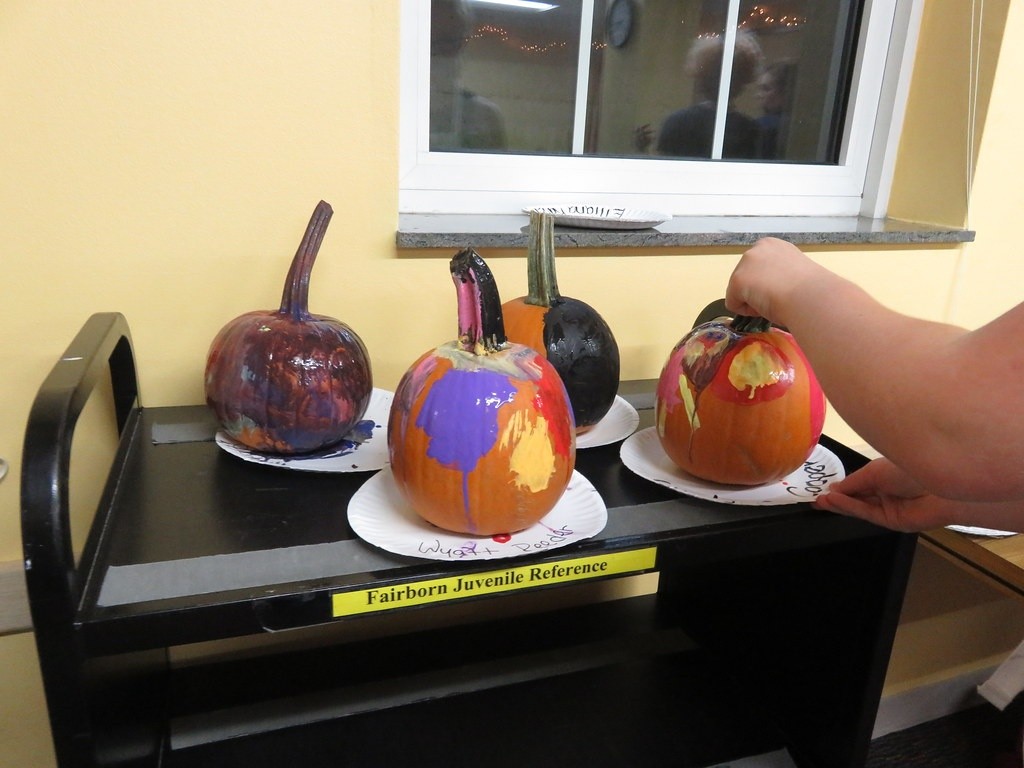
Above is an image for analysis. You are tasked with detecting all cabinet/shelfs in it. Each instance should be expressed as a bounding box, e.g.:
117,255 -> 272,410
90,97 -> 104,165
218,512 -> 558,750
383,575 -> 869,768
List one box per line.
14,310 -> 920,768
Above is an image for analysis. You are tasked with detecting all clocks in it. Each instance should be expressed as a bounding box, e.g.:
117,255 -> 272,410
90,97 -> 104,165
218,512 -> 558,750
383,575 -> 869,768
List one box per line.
606,0 -> 635,48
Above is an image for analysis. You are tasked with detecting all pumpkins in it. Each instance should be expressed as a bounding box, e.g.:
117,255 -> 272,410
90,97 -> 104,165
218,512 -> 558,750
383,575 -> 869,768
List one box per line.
654,313 -> 826,486
204,199 -> 372,454
386,244 -> 577,535
501,211 -> 620,437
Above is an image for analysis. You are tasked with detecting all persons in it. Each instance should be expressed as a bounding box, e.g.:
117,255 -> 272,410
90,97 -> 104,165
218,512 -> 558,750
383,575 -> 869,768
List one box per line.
726,235 -> 1024,534
460,87 -> 508,149
656,32 -> 788,159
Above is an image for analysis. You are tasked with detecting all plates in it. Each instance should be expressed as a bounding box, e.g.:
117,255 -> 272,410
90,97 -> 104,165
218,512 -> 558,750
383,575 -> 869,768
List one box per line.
620,425 -> 846,506
346,464 -> 609,562
523,201 -> 671,230
576,395 -> 640,448
215,387 -> 396,473
